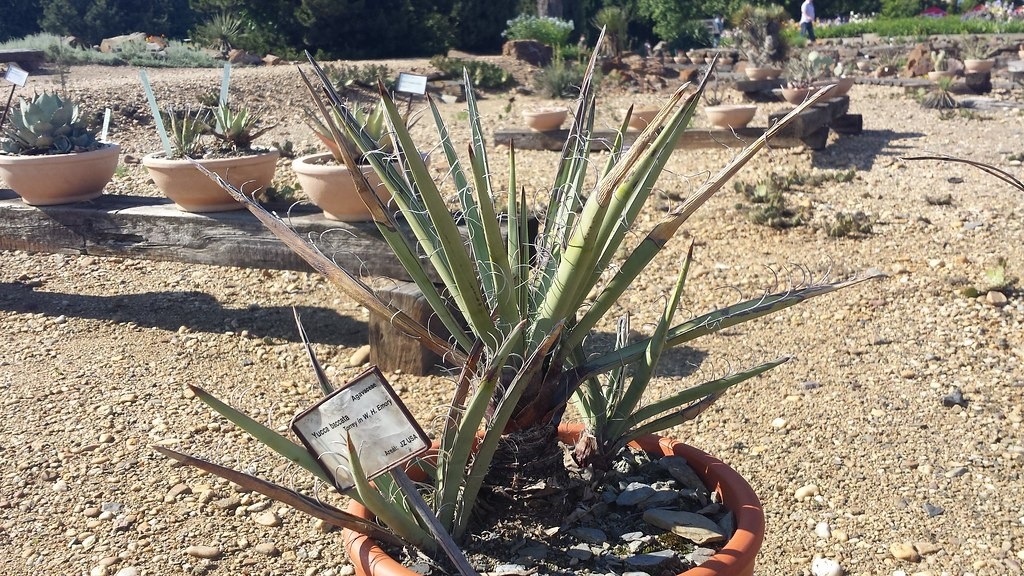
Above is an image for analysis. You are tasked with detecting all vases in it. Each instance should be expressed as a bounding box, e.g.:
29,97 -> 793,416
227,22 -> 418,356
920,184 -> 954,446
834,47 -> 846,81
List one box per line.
522,106 -> 568,131
705,105 -> 758,130
619,104 -> 661,131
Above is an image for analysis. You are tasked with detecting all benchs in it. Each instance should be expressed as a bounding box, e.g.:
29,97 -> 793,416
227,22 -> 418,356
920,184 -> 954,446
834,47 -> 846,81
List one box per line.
767,96 -> 863,150
0,188 -> 539,377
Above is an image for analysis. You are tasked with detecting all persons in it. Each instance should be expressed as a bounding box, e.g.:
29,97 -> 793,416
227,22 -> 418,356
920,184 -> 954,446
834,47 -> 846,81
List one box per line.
799,0 -> 816,44
712,14 -> 724,48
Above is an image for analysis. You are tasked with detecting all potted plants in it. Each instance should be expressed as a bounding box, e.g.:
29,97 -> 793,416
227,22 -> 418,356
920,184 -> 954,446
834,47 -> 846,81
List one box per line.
662,50 -> 732,65
745,47 -> 776,81
152,24 -> 890,576
808,51 -> 842,100
766,62 -> 783,80
962,36 -> 999,73
143,99 -> 279,212
832,66 -> 855,94
779,60 -> 823,108
0,89 -> 121,205
929,50 -> 955,83
291,92 -> 431,221
